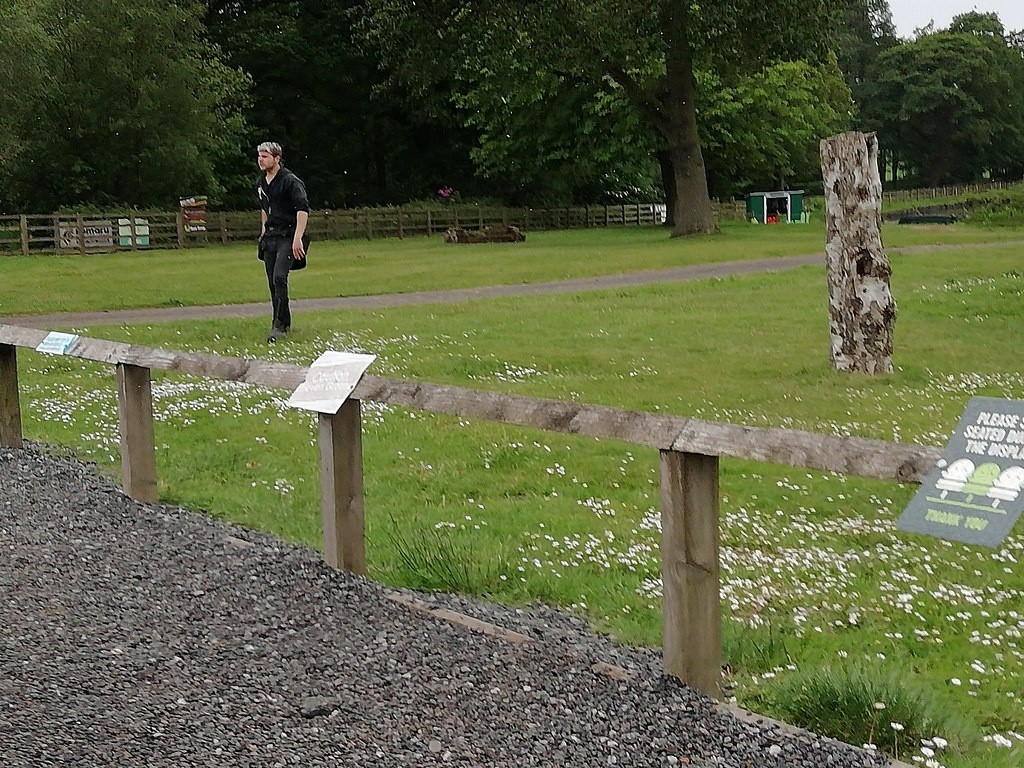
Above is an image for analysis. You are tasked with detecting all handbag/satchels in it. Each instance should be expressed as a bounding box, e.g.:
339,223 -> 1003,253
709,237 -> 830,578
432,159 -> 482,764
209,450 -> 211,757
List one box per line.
257,234 -> 311,270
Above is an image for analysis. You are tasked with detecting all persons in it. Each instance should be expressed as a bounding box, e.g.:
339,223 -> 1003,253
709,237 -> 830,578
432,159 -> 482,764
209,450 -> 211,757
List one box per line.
257,142 -> 308,343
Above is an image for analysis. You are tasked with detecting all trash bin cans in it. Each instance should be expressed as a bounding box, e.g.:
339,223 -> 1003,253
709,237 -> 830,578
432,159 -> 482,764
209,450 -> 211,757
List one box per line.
745,191 -> 804,224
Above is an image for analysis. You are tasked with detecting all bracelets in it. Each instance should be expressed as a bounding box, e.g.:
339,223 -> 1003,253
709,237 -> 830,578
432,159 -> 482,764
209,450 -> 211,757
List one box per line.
261,235 -> 263,238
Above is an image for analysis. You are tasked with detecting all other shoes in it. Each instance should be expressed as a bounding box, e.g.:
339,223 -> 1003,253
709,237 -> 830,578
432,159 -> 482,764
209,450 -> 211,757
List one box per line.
266,329 -> 287,338
